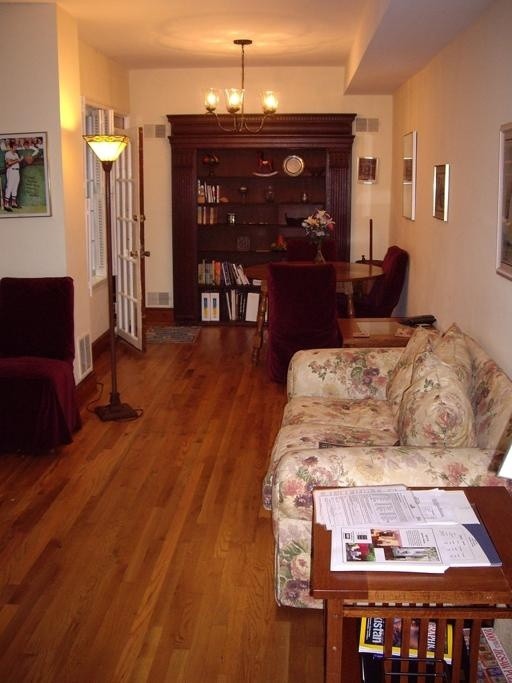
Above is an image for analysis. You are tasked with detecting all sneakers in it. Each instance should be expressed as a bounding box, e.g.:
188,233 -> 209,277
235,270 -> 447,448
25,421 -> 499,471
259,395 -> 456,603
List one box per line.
4,207 -> 13,212
12,204 -> 22,209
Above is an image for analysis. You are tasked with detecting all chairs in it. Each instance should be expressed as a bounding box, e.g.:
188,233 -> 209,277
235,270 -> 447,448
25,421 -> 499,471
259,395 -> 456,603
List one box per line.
264,263 -> 338,379
0,276 -> 83,457
359,245 -> 408,319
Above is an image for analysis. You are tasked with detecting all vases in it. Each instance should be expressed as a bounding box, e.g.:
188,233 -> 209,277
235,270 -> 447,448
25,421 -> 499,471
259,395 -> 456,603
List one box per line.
313,241 -> 325,262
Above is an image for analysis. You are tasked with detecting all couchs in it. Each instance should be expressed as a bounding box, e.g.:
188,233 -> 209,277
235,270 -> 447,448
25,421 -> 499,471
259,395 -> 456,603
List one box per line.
260,323 -> 511,609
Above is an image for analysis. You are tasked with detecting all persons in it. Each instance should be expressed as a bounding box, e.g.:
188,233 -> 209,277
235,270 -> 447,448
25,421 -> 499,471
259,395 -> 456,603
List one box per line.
0,137 -> 43,212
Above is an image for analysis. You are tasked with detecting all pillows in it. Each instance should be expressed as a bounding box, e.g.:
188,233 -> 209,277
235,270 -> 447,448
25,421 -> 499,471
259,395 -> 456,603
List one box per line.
431,322 -> 472,388
398,351 -> 478,449
385,326 -> 441,415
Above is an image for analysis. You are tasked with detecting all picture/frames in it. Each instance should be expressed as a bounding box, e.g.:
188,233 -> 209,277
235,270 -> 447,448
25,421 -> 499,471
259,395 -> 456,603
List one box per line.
494,122 -> 512,280
401,131 -> 416,222
0,131 -> 52,219
433,164 -> 449,223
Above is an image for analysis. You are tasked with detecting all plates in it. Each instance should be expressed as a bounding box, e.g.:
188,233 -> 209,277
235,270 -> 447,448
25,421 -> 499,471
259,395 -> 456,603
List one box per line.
283,156 -> 304,177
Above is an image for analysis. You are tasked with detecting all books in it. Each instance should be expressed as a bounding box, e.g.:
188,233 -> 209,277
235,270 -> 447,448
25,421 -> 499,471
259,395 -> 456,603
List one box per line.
358,614 -> 512,682
394,327 -> 441,338
329,523 -> 502,575
197,178 -> 226,203
197,205 -> 218,224
225,288 -> 247,321
197,258 -> 250,286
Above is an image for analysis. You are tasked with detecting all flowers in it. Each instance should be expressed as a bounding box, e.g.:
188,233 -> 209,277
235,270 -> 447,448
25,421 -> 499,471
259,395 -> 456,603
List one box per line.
301,208 -> 336,241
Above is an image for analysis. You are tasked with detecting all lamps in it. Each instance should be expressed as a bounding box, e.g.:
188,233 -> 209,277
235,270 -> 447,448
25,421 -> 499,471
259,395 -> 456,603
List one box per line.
85,133 -> 139,424
203,39 -> 279,136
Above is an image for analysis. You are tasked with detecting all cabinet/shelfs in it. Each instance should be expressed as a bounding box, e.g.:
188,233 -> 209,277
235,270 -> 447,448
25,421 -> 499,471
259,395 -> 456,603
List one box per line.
164,110 -> 356,325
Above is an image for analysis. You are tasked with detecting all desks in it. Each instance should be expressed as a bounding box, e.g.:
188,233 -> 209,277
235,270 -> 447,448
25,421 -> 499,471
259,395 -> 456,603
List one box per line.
242,261 -> 383,367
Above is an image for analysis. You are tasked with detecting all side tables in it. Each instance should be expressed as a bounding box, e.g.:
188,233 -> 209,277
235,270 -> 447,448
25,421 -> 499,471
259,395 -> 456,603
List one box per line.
310,484 -> 512,683
337,317 -> 442,348
